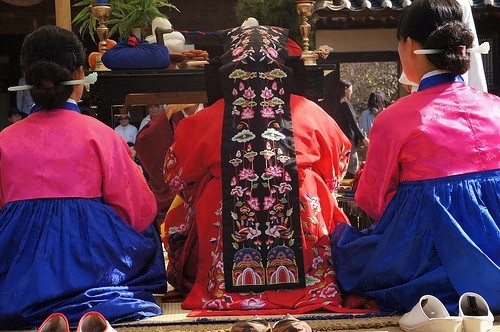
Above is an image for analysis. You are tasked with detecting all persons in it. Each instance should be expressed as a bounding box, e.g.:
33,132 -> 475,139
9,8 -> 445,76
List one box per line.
0,25 -> 164,324
115,103 -> 160,182
157,24 -> 376,317
340,80 -> 391,177
7,73 -> 33,125
328,0 -> 500,313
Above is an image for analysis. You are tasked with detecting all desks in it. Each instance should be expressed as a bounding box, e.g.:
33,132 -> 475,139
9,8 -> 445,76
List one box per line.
87,64 -> 337,129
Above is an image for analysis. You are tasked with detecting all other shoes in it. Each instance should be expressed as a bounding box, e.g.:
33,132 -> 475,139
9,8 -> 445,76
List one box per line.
37,313 -> 70,332
459,292 -> 494,332
271,313 -> 311,332
399,294 -> 464,332
231,315 -> 270,332
76,311 -> 117,332
